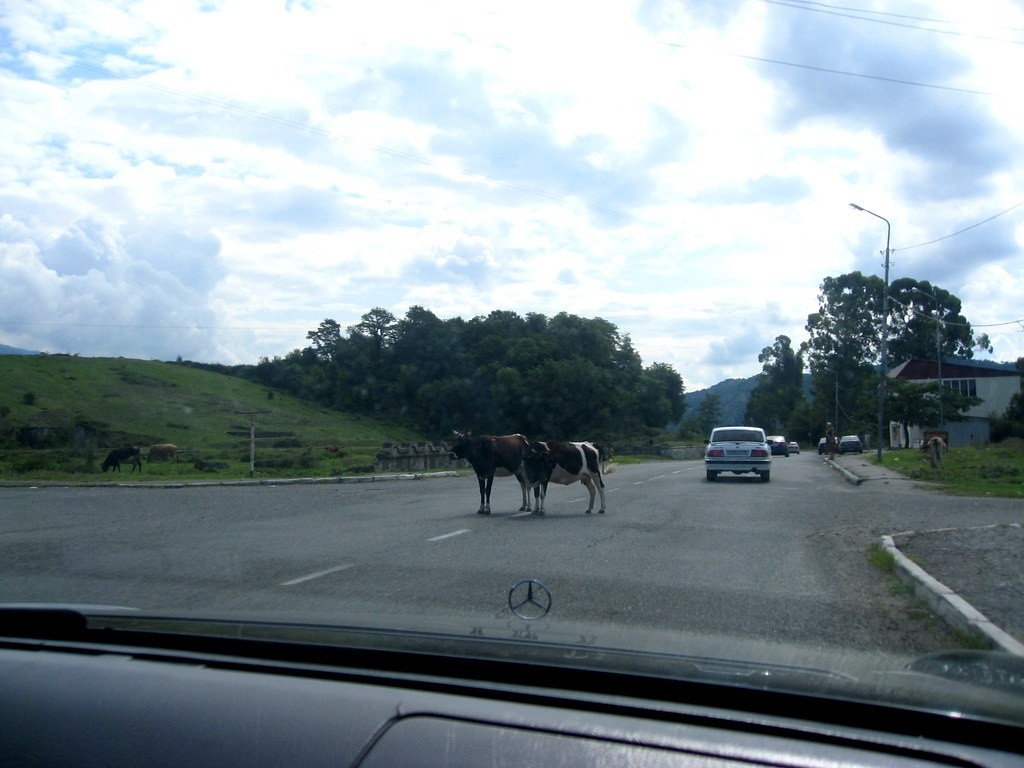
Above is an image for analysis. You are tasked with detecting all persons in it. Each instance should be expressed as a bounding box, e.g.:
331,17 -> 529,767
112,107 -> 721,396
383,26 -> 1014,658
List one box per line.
825,421 -> 837,460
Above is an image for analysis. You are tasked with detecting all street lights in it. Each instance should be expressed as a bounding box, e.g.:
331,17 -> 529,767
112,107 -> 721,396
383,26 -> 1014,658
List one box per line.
849,202 -> 890,465
802,395 -> 812,449
912,287 -> 944,429
234,409 -> 270,478
824,367 -> 839,446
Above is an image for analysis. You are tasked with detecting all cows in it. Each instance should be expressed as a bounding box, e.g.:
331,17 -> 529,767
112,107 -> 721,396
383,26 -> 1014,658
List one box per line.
193,461 -> 231,473
926,436 -> 946,469
100,447 -> 143,475
450,427 -> 615,516
146,444 -> 178,464
325,446 -> 338,454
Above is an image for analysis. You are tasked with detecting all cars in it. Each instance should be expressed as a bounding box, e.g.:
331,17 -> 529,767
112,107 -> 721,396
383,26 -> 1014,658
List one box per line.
703,426 -> 774,481
766,435 -> 790,457
818,437 -> 840,455
838,435 -> 863,454
788,442 -> 800,454
